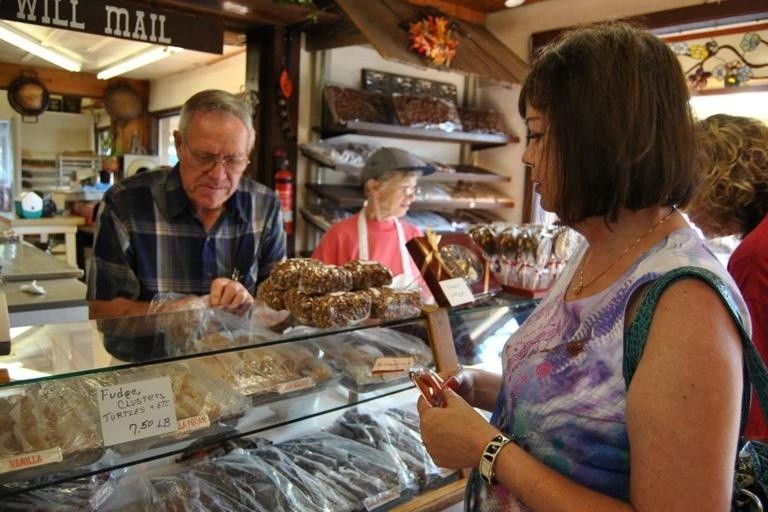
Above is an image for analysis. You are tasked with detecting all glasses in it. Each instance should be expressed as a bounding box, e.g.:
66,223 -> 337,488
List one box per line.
190,155 -> 251,172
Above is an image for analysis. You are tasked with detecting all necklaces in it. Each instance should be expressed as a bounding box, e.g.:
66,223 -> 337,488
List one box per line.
572,209 -> 675,294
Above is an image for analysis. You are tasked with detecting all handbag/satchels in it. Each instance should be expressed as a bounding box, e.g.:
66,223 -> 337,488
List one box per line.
738,434 -> 760,447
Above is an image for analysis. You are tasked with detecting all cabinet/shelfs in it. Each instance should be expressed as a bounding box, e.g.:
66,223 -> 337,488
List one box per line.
300,122 -> 520,257
1,289 -> 541,512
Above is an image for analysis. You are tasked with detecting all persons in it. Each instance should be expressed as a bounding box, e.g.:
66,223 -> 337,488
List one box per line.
410,25 -> 751,511
678,115 -> 768,443
85,89 -> 287,320
310,147 -> 436,291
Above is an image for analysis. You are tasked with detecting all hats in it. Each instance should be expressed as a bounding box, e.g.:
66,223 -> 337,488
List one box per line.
362,148 -> 436,183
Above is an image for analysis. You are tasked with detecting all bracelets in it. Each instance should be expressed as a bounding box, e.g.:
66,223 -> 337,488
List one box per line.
479,430 -> 522,485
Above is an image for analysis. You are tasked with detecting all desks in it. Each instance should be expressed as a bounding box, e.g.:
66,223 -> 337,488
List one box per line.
0,211 -> 86,265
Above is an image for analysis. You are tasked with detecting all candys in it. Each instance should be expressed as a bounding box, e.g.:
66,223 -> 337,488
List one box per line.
440,221 -> 576,289
